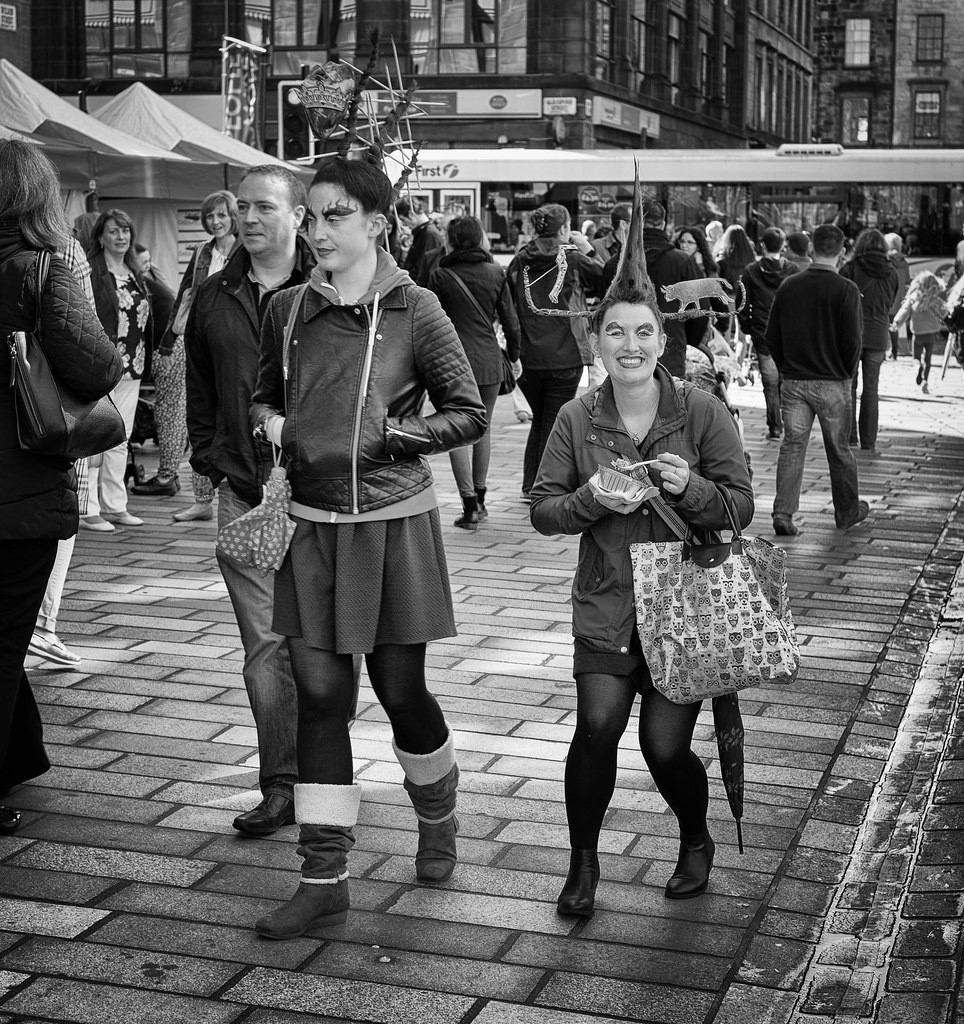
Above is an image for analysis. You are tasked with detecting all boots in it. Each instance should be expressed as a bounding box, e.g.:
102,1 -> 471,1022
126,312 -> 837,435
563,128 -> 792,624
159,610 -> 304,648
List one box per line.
254,783 -> 363,939
392,720 -> 460,881
475,486 -> 487,517
454,496 -> 479,530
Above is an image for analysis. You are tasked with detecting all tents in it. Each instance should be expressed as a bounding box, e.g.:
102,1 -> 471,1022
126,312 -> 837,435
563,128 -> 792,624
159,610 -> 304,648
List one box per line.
0,43 -> 319,201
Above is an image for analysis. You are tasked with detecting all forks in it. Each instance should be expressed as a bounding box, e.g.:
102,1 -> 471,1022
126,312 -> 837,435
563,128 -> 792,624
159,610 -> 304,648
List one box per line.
611,453 -> 680,470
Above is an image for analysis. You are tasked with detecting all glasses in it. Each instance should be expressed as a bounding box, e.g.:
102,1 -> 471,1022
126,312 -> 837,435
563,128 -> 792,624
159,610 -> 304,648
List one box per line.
678,239 -> 697,244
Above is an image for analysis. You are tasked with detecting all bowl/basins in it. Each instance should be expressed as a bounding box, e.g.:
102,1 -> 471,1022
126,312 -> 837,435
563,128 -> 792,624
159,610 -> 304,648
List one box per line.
595,463 -> 649,499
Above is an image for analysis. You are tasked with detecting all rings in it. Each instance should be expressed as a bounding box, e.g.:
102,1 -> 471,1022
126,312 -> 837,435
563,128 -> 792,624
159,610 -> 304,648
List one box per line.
674,467 -> 677,475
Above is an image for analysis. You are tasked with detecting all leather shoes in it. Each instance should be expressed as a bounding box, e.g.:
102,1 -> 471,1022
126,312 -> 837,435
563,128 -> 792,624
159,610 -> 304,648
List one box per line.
557,848 -> 600,916
232,794 -> 296,833
665,829 -> 715,900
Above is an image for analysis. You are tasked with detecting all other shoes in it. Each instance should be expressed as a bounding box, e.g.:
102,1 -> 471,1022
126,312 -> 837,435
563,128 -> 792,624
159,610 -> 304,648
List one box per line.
520,493 -> 532,502
773,519 -> 797,536
100,513 -> 144,525
27,630 -> 82,665
766,431 -> 780,441
79,518 -> 115,531
836,501 -> 869,528
173,506 -> 213,521
0,808 -> 21,829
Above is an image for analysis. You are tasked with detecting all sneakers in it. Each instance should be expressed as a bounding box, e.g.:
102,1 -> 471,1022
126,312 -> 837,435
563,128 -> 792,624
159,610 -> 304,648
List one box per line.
174,476 -> 181,490
132,478 -> 177,495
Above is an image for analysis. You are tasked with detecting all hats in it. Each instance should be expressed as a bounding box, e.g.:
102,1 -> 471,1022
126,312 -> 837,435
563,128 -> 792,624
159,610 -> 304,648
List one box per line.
884,232 -> 901,248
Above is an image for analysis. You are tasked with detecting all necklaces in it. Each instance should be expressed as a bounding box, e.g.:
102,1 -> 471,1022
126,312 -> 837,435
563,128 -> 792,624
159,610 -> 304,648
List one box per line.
617,397 -> 659,446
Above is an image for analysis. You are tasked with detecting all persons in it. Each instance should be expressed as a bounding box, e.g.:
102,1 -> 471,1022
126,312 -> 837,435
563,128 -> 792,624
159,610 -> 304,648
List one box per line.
56,188 -> 964,530
183,30 -> 489,940
531,156 -> 803,922
0,133 -> 124,840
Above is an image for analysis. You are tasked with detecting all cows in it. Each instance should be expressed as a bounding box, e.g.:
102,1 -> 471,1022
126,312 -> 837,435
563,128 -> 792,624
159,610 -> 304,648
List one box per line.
661,278 -> 736,313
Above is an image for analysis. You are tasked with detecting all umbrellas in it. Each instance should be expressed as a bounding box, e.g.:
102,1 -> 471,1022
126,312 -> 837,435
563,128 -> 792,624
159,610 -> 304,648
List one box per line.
215,467 -> 297,578
712,483 -> 746,855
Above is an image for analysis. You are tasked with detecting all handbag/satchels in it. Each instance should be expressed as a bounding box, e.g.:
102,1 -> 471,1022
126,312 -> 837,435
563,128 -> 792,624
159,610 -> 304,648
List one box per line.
629,487 -> 800,705
171,241 -> 211,335
497,348 -> 515,395
7,247 -> 127,458
